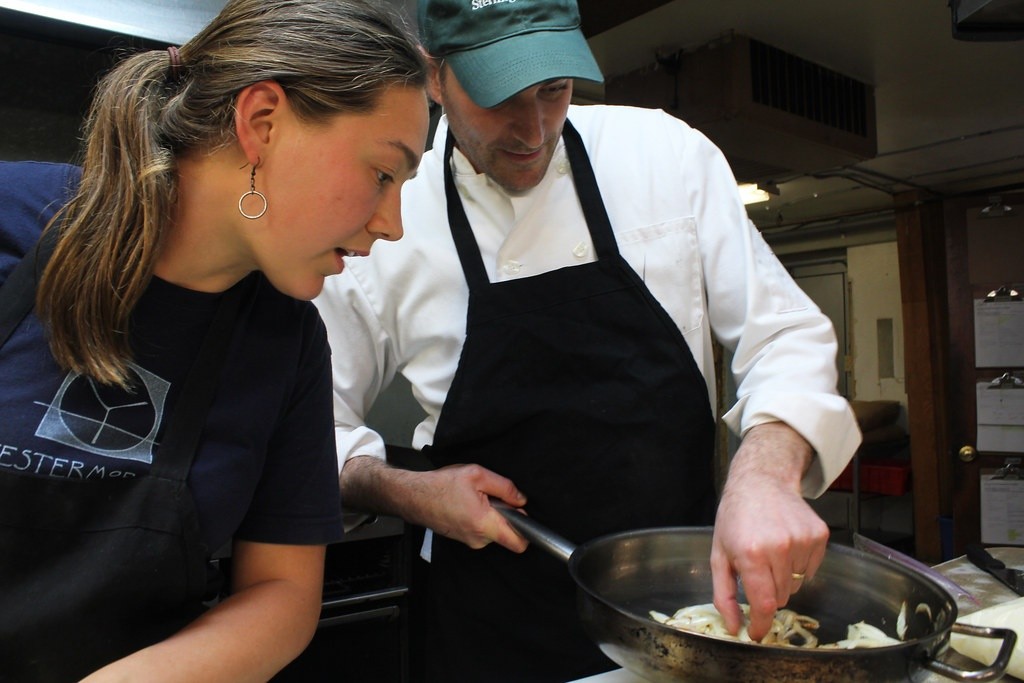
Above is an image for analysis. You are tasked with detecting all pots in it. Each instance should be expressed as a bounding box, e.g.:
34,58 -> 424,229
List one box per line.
488,495 -> 1018,683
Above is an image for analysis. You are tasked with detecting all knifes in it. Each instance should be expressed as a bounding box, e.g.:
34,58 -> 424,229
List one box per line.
966,546 -> 1024,597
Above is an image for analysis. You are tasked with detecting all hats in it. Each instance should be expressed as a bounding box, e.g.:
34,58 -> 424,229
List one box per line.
416,1 -> 605,103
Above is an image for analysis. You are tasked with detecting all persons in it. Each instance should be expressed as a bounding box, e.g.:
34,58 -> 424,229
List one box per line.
1,0 -> 431,683
311,1 -> 863,683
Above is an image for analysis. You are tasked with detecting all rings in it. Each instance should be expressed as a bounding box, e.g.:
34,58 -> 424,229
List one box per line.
791,572 -> 806,579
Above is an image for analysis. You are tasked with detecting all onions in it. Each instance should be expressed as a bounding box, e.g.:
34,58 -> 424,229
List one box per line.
650,600 -> 909,648
